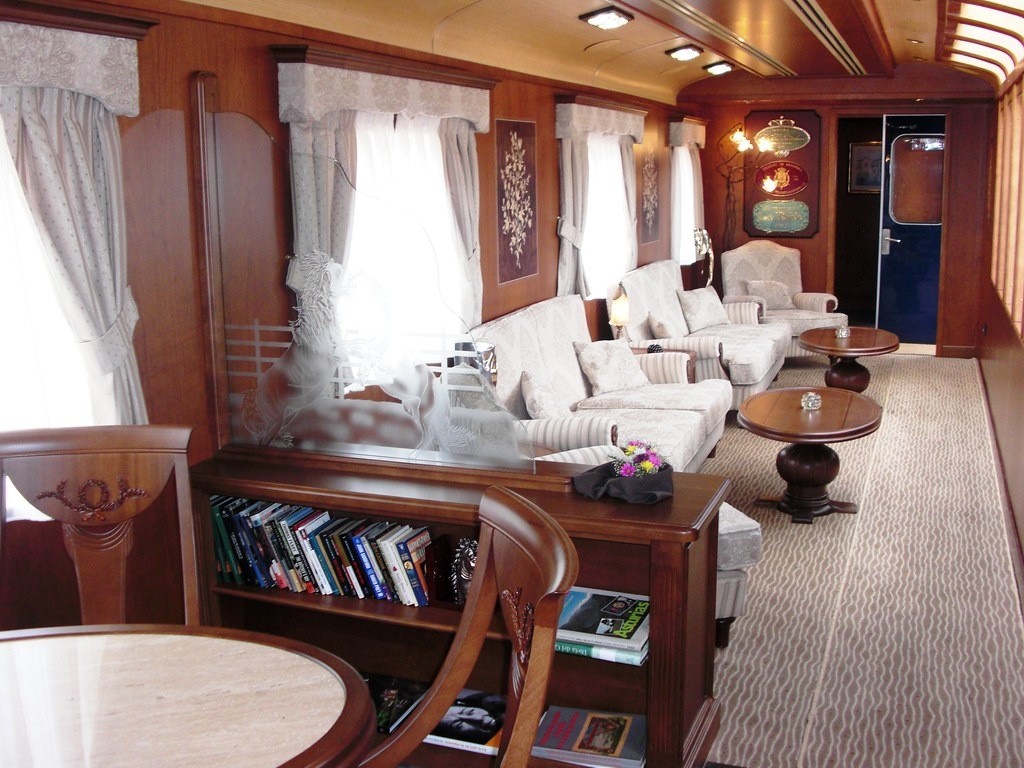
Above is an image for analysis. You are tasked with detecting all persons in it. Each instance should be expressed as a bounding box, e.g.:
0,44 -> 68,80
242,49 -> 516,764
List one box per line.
440,705 -> 496,735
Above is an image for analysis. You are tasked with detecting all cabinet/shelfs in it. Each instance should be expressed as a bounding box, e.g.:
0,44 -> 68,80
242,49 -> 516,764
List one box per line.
187,443 -> 731,768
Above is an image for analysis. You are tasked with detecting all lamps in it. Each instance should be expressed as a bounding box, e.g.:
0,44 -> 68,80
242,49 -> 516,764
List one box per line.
578,5 -> 634,30
702,60 -> 735,75
665,44 -> 704,61
716,122 -> 779,251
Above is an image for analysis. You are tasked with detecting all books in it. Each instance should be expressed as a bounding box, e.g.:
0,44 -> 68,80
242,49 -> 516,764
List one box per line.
553,585 -> 650,667
360,669 -> 507,756
530,703 -> 646,768
209,495 -> 478,607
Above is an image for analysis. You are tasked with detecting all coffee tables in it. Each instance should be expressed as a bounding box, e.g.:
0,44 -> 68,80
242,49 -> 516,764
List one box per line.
735,386 -> 884,525
797,326 -> 899,394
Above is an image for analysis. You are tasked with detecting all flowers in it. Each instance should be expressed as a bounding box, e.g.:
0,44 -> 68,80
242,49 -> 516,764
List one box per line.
607,439 -> 674,478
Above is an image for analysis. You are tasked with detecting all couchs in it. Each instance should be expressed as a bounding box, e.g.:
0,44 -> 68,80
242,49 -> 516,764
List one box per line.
721,240 -> 848,367
605,259 -> 792,429
465,293 -> 733,473
432,362 -> 761,650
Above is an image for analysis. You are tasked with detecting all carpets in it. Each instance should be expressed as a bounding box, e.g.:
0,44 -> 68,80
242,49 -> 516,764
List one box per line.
695,339 -> 1024,768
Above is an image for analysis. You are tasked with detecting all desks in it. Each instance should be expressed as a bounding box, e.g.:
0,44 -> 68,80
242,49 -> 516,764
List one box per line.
0,623 -> 378,768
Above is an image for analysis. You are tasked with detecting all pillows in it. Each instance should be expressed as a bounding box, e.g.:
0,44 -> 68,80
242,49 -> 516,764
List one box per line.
745,280 -> 797,310
521,370 -> 573,419
572,335 -> 651,397
648,311 -> 683,339
675,286 -> 731,334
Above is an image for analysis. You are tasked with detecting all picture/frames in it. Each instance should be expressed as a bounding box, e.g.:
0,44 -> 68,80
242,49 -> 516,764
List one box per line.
846,139 -> 882,194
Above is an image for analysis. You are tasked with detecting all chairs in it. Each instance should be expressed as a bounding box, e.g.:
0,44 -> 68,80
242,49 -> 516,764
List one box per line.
359,482 -> 579,768
0,423 -> 202,633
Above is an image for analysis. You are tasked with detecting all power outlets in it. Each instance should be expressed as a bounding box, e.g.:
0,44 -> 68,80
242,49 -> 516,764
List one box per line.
982,320 -> 987,336
